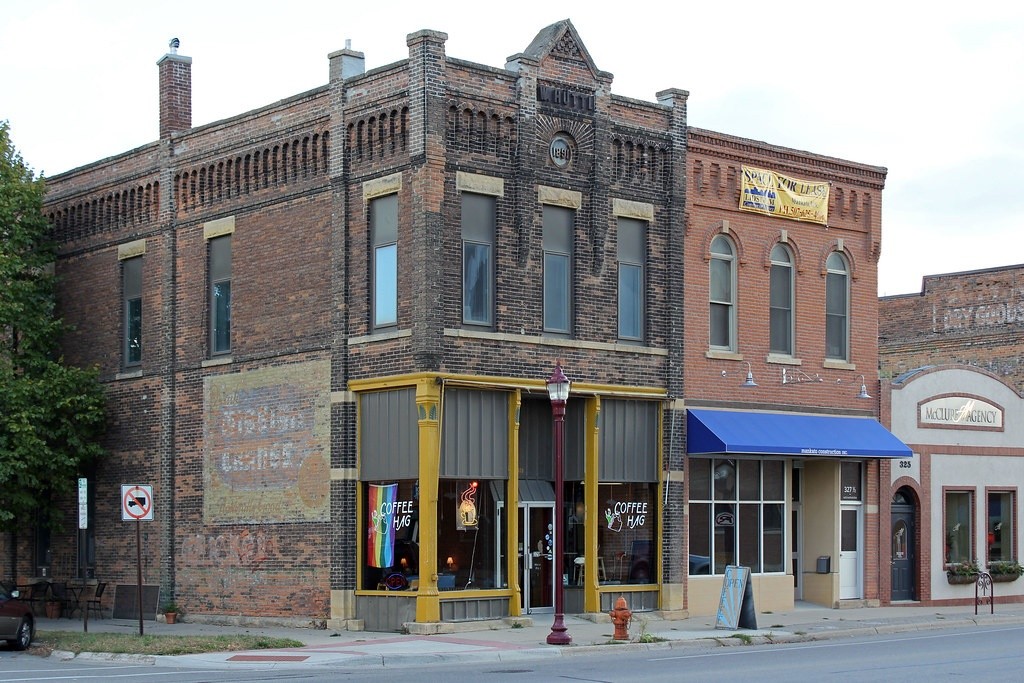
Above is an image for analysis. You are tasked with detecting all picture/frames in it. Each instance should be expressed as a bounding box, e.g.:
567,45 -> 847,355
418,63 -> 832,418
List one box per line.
949,575 -> 978,585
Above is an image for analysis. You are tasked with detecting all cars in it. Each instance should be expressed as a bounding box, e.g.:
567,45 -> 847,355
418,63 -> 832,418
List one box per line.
0,581 -> 35,651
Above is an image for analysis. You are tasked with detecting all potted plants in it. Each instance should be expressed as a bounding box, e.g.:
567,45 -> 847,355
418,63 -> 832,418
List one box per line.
946,534 -> 954,553
161,603 -> 177,624
989,561 -> 1024,582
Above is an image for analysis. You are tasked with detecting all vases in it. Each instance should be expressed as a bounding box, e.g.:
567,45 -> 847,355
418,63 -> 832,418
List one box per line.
45,602 -> 61,618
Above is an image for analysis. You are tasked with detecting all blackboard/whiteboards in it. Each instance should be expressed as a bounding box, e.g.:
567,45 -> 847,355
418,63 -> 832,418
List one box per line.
112,583 -> 160,621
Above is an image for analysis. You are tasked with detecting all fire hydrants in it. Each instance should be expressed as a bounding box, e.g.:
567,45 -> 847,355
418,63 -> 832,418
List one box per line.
610,596 -> 632,640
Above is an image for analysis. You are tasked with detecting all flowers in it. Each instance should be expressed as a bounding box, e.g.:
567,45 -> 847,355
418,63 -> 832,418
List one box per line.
949,560 -> 982,577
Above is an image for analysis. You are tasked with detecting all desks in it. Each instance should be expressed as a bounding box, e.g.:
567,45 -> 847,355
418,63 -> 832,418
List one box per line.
409,575 -> 456,593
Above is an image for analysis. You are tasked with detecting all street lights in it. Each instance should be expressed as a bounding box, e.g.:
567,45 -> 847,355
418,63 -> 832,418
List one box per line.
545,360 -> 572,644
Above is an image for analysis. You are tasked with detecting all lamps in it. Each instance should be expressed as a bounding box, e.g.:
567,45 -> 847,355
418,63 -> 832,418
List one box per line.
722,360 -> 757,388
836,373 -> 874,399
446,557 -> 454,572
401,558 -> 407,568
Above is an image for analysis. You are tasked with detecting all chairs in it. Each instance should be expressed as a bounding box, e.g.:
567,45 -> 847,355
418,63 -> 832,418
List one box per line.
12,581 -> 107,621
